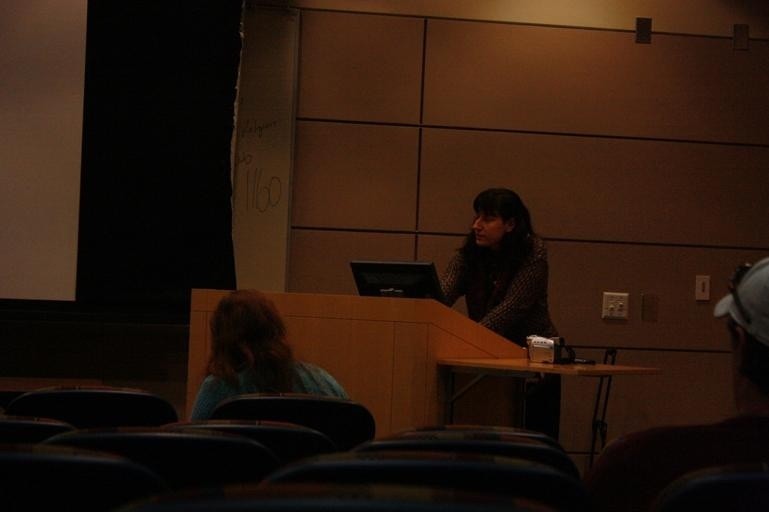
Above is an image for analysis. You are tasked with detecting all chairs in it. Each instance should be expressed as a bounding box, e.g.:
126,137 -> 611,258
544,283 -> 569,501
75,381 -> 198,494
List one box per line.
0,382 -> 769,511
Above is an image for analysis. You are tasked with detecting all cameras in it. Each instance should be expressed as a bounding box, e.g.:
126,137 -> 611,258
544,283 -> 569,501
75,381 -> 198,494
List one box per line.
527,335 -> 564,364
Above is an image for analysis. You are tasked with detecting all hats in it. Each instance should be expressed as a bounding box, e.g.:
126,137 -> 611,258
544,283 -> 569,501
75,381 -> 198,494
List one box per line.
713,257 -> 769,346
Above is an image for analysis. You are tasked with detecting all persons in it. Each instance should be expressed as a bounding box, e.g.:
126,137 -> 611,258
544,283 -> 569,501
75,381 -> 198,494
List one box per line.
439,184 -> 560,347
187,287 -> 355,421
582,255 -> 769,512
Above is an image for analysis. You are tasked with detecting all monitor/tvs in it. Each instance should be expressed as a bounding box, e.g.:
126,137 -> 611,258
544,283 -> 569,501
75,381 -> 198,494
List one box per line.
350,261 -> 448,306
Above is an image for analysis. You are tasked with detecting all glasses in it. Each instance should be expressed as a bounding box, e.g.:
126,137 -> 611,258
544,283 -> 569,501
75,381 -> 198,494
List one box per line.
728,263 -> 752,324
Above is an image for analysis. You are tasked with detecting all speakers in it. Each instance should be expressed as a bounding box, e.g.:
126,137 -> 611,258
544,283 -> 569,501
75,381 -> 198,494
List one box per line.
636,18 -> 652,43
732,23 -> 749,50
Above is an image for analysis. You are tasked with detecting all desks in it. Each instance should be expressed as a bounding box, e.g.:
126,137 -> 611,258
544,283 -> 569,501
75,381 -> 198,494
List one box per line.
438,355 -> 664,475
